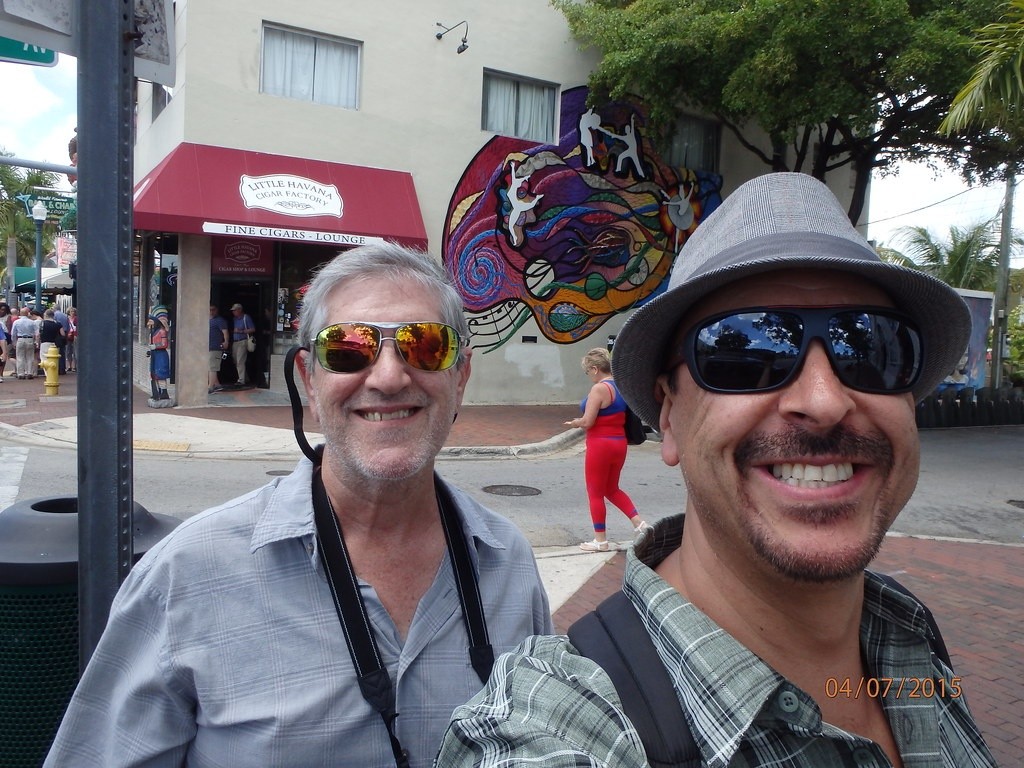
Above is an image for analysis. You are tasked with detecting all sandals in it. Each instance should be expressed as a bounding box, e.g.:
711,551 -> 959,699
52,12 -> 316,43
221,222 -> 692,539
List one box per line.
579,538 -> 609,551
633,521 -> 650,543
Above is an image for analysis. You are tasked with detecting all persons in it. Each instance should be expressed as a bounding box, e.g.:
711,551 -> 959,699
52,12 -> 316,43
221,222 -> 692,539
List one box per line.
208,301 -> 229,395
40,310 -> 65,348
51,304 -> 69,374
65,306 -> 77,373
230,303 -> 255,387
41,240 -> 555,767
562,346 -> 653,552
146,304 -> 170,401
430,171 -> 999,767
0,302 -> 44,384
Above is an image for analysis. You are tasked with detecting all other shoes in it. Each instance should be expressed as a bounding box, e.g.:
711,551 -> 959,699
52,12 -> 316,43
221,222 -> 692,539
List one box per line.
67,368 -> 71,371
27,374 -> 33,378
209,385 -> 223,394
0,376 -> 3,382
19,376 -> 25,379
72,368 -> 76,372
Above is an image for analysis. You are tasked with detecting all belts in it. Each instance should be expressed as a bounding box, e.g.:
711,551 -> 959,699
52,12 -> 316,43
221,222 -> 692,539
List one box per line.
41,340 -> 55,343
17,336 -> 33,338
233,338 -> 247,342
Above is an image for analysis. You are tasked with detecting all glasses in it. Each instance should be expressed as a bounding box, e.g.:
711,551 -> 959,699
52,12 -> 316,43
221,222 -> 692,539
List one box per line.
669,303 -> 926,394
311,321 -> 465,373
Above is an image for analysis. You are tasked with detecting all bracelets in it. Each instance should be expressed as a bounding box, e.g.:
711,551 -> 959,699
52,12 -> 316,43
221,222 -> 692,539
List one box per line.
241,329 -> 243,333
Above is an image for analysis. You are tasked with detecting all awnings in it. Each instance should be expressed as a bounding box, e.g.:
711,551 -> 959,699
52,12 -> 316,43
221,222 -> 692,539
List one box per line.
34,271 -> 76,296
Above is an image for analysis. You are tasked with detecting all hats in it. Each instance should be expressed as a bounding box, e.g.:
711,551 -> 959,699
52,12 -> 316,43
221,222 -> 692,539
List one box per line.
609,171 -> 972,435
230,303 -> 243,311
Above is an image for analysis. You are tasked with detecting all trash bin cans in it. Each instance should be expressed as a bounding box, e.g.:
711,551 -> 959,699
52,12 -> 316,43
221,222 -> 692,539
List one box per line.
0,498 -> 183,767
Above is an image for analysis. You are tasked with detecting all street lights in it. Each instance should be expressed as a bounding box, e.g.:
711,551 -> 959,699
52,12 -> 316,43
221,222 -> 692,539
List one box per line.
32,201 -> 47,312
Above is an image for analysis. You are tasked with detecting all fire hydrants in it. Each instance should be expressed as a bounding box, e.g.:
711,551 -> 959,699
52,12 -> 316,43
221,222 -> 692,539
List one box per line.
39,344 -> 61,397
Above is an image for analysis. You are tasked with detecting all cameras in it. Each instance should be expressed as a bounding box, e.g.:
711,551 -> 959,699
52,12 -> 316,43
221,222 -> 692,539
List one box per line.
222,353 -> 227,359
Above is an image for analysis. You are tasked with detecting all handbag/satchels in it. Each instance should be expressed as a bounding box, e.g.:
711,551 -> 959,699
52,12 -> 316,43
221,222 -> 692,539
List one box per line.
248,336 -> 256,352
624,405 -> 648,445
